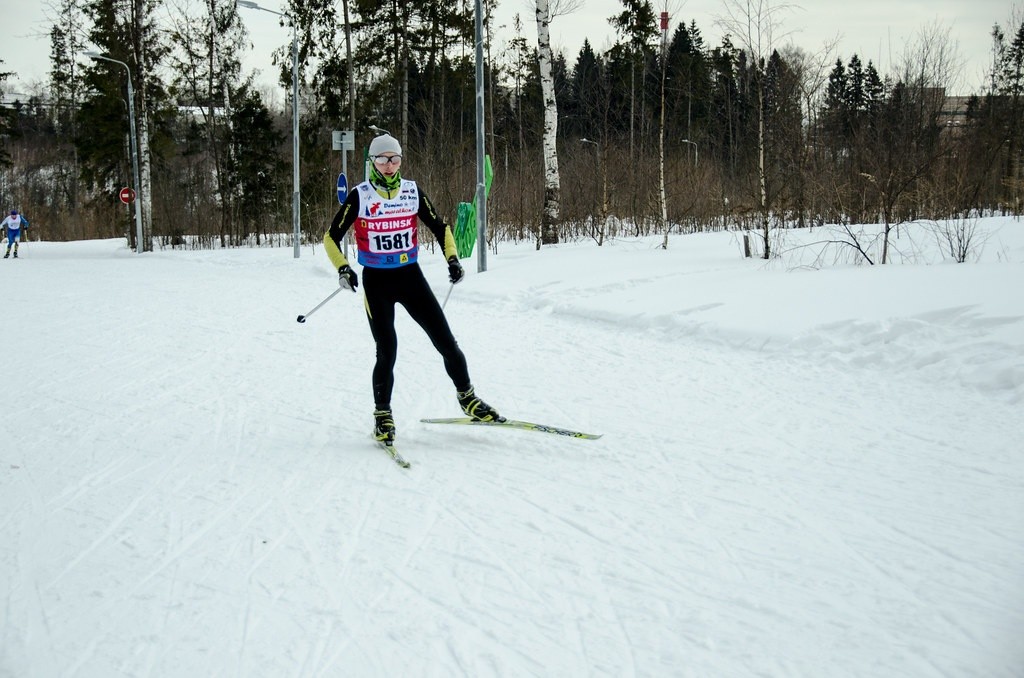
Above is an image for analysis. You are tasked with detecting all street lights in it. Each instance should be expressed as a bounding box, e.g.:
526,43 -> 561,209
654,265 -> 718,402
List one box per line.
579,138 -> 599,168
82,50 -> 144,253
236,0 -> 301,257
486,133 -> 509,189
682,139 -> 697,165
88,93 -> 132,247
369,125 -> 391,136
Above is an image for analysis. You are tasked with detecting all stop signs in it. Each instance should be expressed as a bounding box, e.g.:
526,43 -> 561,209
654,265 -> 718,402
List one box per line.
119,187 -> 136,204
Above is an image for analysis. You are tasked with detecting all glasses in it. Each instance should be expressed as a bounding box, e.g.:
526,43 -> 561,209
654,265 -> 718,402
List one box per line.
370,154 -> 402,165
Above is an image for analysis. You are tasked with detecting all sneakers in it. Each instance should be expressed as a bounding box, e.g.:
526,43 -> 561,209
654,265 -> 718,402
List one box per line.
373,408 -> 395,441
457,386 -> 499,421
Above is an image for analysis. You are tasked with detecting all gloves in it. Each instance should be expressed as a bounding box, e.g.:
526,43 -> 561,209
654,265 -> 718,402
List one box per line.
24,225 -> 28,230
1,228 -> 4,230
448,255 -> 465,285
338,265 -> 358,292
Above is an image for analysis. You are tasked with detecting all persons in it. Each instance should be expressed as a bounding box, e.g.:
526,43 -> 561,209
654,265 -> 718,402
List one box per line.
0,211 -> 28,259
323,135 -> 499,440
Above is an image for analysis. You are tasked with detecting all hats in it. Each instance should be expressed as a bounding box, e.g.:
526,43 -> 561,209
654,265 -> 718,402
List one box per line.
11,210 -> 17,215
369,134 -> 402,162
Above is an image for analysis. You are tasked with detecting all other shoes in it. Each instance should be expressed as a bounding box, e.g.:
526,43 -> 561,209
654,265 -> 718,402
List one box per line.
14,252 -> 18,257
4,253 -> 10,258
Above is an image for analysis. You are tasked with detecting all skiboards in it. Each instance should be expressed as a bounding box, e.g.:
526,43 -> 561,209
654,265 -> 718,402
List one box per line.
369,416 -> 604,470
3,256 -> 24,260
